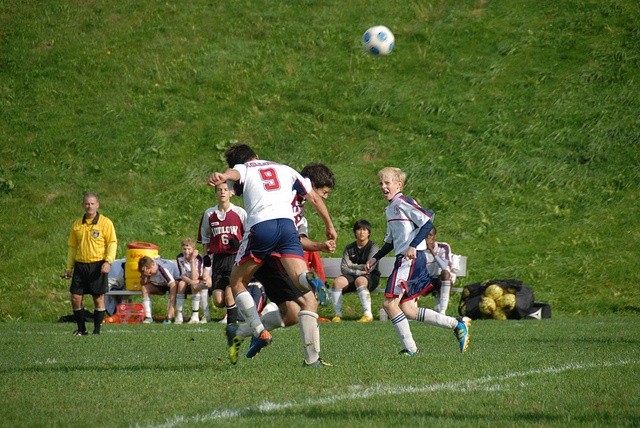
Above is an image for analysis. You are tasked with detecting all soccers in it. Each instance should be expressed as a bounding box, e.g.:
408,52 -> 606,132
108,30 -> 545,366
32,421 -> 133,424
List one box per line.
479,297 -> 496,314
507,286 -> 516,293
484,285 -> 503,299
499,294 -> 516,312
493,306 -> 507,320
459,300 -> 466,316
481,279 -> 490,286
496,296 -> 503,306
362,24 -> 395,57
463,288 -> 471,299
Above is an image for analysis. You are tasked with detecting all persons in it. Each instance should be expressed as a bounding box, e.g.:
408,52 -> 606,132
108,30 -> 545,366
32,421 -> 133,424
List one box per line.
174,237 -> 204,324
64,192 -> 117,336
224,163 -> 335,366
424,226 -> 457,316
332,219 -> 380,323
138,256 -> 180,324
363,166 -> 472,361
197,181 -> 248,325
207,144 -> 338,370
302,249 -> 326,283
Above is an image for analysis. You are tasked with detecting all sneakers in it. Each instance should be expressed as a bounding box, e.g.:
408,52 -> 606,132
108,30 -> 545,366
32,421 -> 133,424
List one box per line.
357,314 -> 373,322
398,348 -> 421,356
226,323 -> 244,363
302,357 -> 337,367
306,270 -> 331,306
246,328 -> 273,357
332,313 -> 342,322
454,315 -> 472,352
